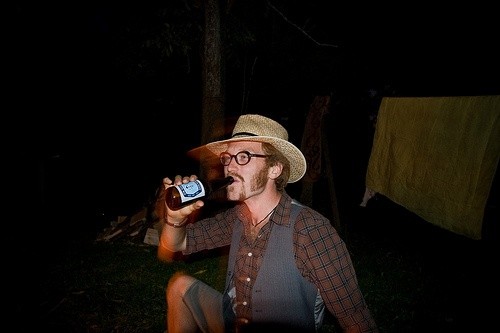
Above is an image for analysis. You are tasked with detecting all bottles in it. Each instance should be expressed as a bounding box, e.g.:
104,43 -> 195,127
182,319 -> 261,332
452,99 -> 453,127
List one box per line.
165,176 -> 234,211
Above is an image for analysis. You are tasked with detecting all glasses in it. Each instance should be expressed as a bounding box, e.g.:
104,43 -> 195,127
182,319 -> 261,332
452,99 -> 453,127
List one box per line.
220,150 -> 272,166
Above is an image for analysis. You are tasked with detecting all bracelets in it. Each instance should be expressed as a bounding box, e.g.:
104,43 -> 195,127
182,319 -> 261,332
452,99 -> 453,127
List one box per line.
164,211 -> 188,228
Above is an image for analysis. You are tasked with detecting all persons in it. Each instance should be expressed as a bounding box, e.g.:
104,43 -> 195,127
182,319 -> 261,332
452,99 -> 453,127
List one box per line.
157,114 -> 378,333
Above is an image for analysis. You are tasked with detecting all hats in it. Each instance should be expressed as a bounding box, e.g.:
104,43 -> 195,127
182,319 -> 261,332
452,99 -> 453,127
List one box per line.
206,114 -> 306,183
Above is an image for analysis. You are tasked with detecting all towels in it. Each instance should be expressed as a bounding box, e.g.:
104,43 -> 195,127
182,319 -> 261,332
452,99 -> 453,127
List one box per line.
360,95 -> 500,241
